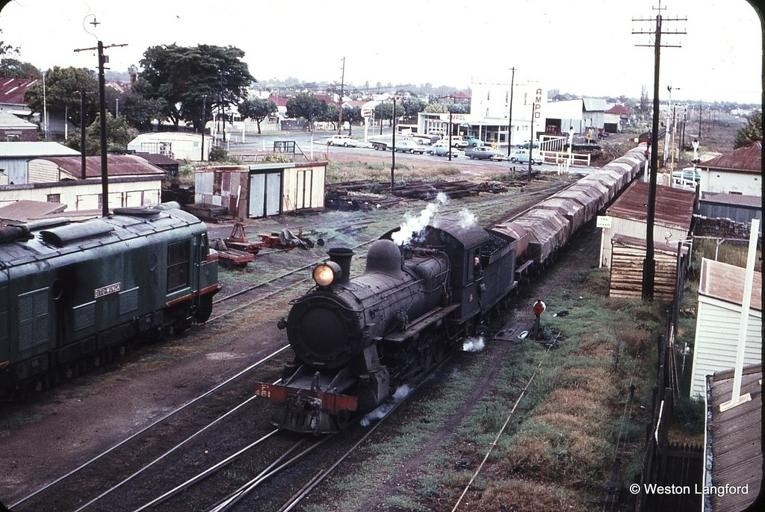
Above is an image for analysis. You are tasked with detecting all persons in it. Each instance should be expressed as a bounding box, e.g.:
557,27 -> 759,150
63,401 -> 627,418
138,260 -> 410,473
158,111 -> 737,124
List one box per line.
474,255 -> 480,267
533,300 -> 547,320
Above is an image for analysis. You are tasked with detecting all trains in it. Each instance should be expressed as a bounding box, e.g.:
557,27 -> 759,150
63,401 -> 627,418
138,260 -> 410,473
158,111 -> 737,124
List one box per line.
0,200 -> 223,414
638,120 -> 683,148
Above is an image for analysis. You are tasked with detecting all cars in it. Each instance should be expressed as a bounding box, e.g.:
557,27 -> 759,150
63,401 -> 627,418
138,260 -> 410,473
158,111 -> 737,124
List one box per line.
367,124 -> 544,166
326,135 -> 359,148
